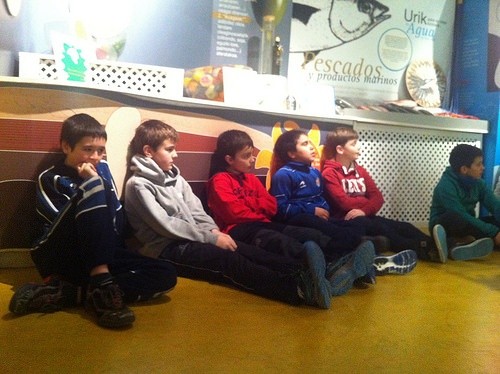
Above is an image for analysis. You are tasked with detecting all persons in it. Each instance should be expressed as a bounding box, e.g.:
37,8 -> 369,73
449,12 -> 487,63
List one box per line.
7,114 -> 177,330
125,120 -> 376,310
207,128 -> 448,289
429,143 -> 500,261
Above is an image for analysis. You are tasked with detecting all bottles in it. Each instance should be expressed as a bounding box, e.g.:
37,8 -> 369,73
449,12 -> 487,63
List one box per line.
271,36 -> 282,75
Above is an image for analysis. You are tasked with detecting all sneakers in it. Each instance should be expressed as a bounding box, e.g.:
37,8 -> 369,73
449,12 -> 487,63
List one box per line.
294,240 -> 330,309
326,239 -> 375,296
8,272 -> 70,316
449,237 -> 494,260
356,270 -> 377,286
429,224 -> 448,263
84,276 -> 136,327
373,249 -> 417,276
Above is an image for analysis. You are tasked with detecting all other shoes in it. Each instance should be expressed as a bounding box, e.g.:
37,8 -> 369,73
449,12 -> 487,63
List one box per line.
360,235 -> 390,253
